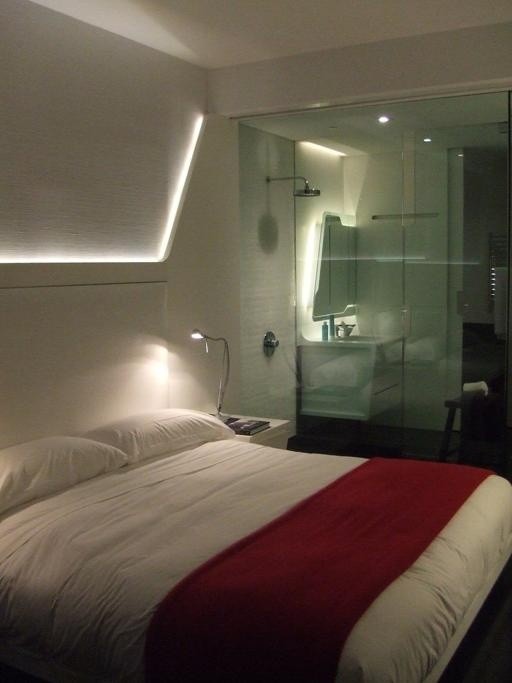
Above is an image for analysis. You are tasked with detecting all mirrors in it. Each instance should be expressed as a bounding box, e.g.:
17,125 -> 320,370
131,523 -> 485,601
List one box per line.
309,210 -> 357,320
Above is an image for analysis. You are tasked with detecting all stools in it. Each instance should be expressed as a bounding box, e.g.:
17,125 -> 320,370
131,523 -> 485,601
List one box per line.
438,390 -> 500,471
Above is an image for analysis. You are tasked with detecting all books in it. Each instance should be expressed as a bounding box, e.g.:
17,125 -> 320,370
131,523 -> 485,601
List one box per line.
209,413 -> 270,435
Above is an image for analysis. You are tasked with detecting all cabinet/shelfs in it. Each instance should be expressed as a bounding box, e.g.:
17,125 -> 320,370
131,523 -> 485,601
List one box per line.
299,341 -> 404,422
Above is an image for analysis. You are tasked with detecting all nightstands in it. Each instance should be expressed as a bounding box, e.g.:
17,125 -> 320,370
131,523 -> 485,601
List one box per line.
209,410 -> 291,449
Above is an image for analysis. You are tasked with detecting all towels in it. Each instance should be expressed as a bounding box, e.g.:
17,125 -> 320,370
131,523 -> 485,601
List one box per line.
492,265 -> 509,339
462,381 -> 488,397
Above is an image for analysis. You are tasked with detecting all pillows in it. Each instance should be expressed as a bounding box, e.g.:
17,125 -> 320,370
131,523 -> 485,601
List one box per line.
0,434 -> 131,515
80,408 -> 236,467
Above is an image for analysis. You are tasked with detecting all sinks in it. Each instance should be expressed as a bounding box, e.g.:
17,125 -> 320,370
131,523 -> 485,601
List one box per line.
339,336 -> 384,342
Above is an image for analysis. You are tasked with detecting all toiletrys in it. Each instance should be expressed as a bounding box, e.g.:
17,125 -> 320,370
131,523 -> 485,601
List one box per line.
322,322 -> 328,341
330,316 -> 334,340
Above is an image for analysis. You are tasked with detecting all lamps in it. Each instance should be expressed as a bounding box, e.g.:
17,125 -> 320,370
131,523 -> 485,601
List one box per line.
188,328 -> 234,426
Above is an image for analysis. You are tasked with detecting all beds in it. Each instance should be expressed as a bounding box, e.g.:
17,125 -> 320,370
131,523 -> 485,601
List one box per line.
1,405 -> 511,682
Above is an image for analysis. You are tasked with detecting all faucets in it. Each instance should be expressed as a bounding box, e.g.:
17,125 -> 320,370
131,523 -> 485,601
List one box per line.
345,327 -> 352,334
